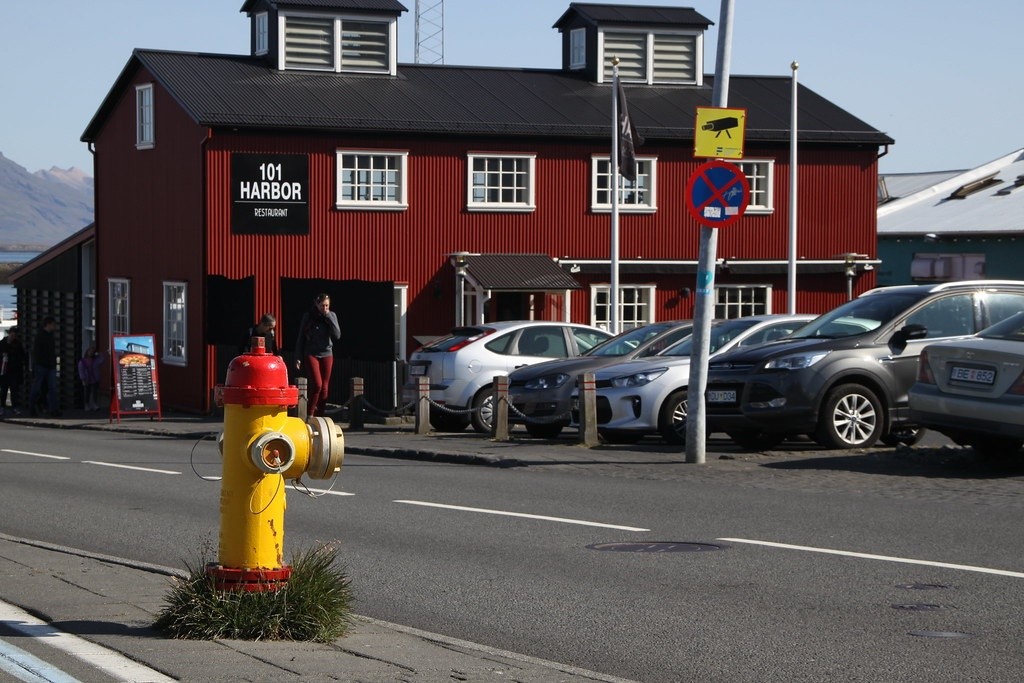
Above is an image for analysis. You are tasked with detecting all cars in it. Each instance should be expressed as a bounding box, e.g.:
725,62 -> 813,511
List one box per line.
565,313 -> 927,447
906,311 -> 1023,464
506,316 -> 720,441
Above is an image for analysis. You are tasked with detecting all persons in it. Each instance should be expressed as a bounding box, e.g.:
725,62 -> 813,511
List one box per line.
78,346 -> 109,413
0,327 -> 26,418
27,315 -> 64,419
236,314 -> 287,362
293,293 -> 342,420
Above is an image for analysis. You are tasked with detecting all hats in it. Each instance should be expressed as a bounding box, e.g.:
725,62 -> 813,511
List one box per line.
5,326 -> 20,334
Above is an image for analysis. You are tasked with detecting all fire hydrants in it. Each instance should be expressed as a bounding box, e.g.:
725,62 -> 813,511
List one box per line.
205,336 -> 345,594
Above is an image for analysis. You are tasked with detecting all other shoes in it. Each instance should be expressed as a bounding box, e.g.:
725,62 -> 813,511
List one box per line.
85,407 -> 91,412
13,409 -> 21,415
0,407 -> 5,416
92,404 -> 100,412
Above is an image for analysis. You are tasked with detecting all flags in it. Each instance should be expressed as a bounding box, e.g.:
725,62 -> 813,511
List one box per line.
615,72 -> 640,183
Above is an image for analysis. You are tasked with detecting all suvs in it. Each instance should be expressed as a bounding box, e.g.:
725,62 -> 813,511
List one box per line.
403,319 -> 640,436
703,280 -> 1024,451
